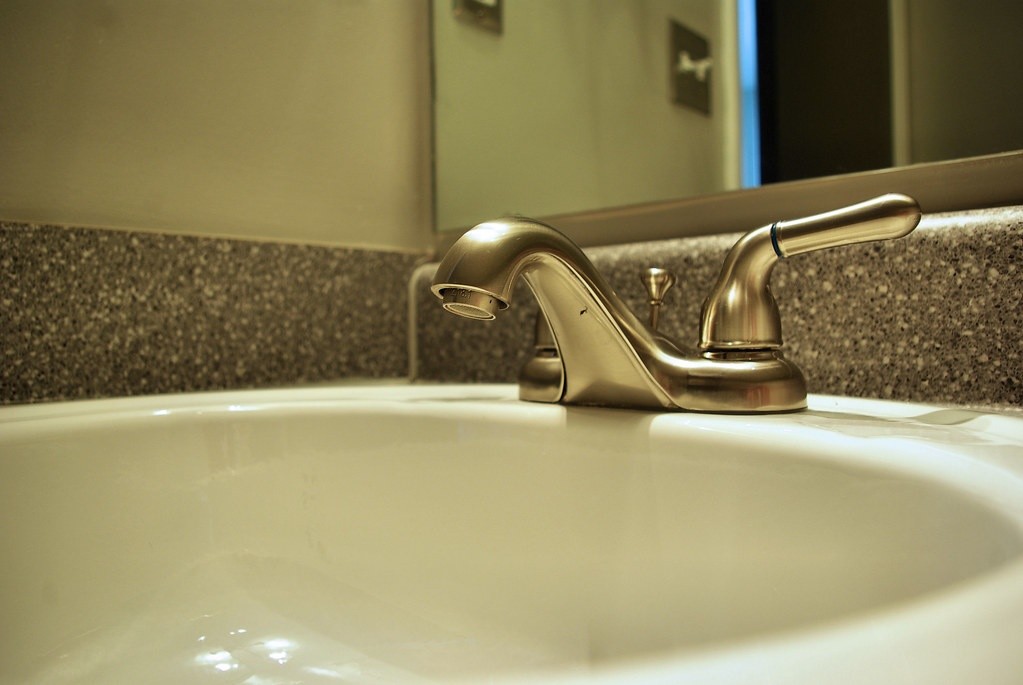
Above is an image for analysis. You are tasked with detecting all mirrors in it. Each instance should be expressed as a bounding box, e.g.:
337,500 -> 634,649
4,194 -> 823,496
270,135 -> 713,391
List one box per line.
413,0 -> 1023,257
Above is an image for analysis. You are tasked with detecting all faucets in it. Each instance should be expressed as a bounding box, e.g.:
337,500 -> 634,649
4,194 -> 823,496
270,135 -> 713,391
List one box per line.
430,193 -> 921,415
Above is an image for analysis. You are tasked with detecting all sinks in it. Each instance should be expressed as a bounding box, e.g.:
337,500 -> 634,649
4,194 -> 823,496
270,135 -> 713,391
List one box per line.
2,378 -> 1022,685
